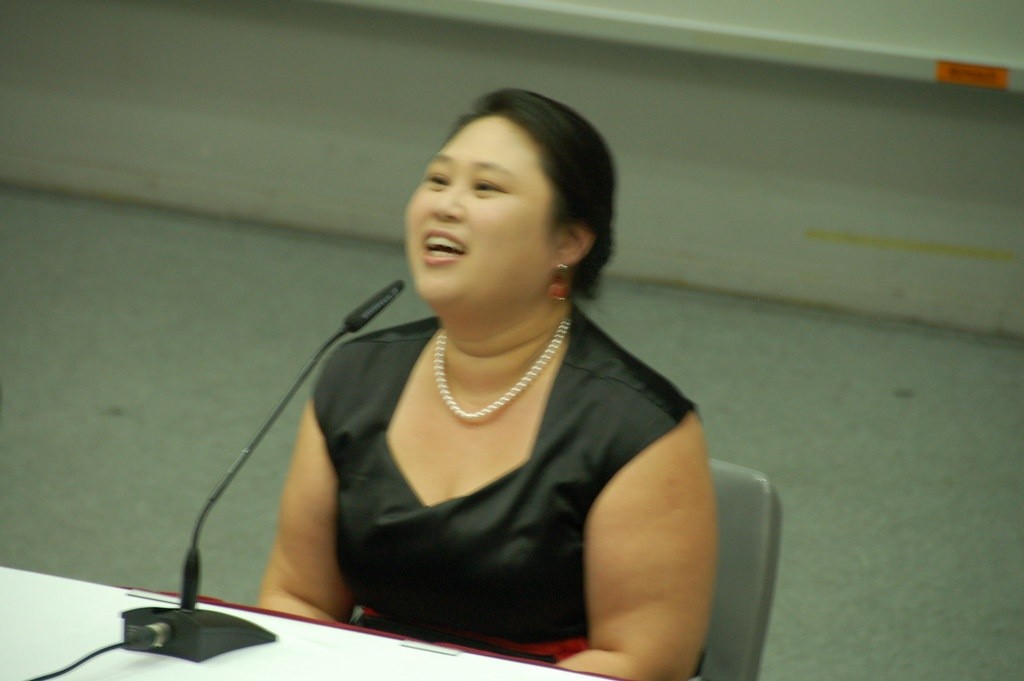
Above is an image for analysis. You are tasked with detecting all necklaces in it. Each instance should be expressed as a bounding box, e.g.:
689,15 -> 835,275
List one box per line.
434,317 -> 571,420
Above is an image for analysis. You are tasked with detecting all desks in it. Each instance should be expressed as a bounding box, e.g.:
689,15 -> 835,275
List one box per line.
0,566 -> 627,681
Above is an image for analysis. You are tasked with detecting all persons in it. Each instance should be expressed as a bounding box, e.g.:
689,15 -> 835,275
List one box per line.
257,87 -> 718,681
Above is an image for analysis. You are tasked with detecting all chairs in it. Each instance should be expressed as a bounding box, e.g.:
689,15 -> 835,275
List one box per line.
693,454 -> 782,680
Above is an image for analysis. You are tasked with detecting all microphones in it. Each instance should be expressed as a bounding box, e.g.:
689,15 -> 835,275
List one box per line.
120,278 -> 405,662
